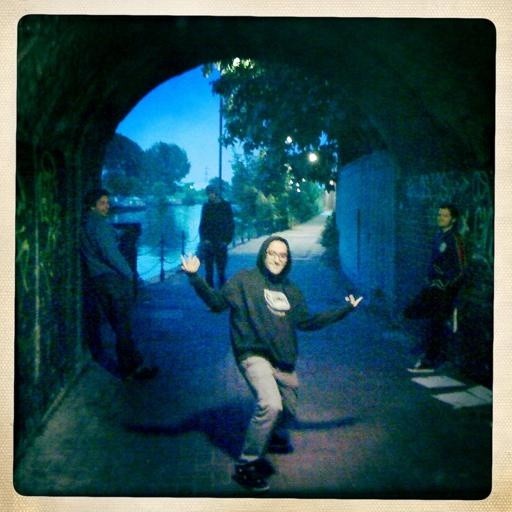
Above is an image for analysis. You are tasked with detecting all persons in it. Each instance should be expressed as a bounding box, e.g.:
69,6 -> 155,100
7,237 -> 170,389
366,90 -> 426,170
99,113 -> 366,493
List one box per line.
199,185 -> 235,290
79,188 -> 163,384
404,204 -> 466,374
181,235 -> 363,493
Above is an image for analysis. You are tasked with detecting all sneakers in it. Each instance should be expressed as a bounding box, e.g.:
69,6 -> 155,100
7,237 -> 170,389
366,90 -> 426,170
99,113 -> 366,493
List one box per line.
232,460 -> 269,490
408,360 -> 434,372
